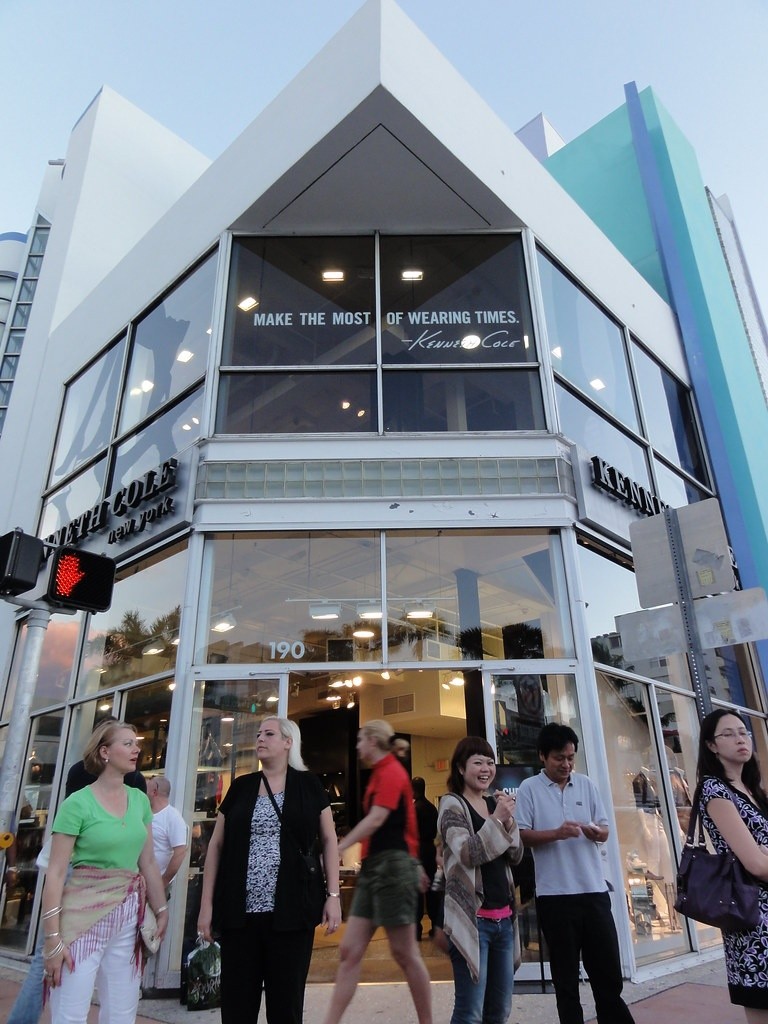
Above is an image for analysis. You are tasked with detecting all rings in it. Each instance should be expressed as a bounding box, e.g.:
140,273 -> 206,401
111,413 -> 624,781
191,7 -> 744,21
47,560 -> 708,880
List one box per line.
44,970 -> 48,976
334,925 -> 338,928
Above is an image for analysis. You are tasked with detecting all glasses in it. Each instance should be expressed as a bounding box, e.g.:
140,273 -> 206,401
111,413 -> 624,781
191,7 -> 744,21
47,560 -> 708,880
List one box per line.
149,775 -> 158,790
711,730 -> 752,741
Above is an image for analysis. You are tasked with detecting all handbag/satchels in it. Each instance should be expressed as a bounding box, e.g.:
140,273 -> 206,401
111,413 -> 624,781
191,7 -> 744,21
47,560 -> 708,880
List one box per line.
186,936 -> 222,1011
138,900 -> 161,960
297,849 -> 327,918
674,776 -> 760,929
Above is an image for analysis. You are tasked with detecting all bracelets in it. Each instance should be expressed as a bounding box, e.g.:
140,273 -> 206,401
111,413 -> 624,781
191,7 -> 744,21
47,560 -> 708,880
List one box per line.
41,907 -> 63,919
327,893 -> 340,897
43,941 -> 64,959
497,819 -> 503,827
155,905 -> 168,918
44,932 -> 60,938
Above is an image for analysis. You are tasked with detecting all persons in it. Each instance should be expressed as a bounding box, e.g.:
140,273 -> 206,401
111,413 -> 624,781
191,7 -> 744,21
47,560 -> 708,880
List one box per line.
668,766 -> 694,848
632,766 -> 684,930
514,722 -> 635,1024
0,836 -> 72,1024
436,737 -> 524,1024
43,721 -> 170,1024
411,776 -> 438,941
198,716 -> 342,1024
323,719 -> 433,1024
144,775 -> 187,965
67,716 -> 147,798
697,709 -> 768,1024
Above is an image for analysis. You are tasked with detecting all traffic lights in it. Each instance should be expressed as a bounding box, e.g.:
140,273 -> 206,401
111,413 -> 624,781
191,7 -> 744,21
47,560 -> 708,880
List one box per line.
47,547 -> 117,617
0,530 -> 45,599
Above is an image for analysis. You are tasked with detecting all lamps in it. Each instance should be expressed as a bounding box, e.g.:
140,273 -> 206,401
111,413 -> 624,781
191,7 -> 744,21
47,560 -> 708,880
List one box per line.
210,615 -> 235,635
326,674 -> 362,710
142,632 -> 179,656
306,598 -> 434,640
290,683 -> 299,698
